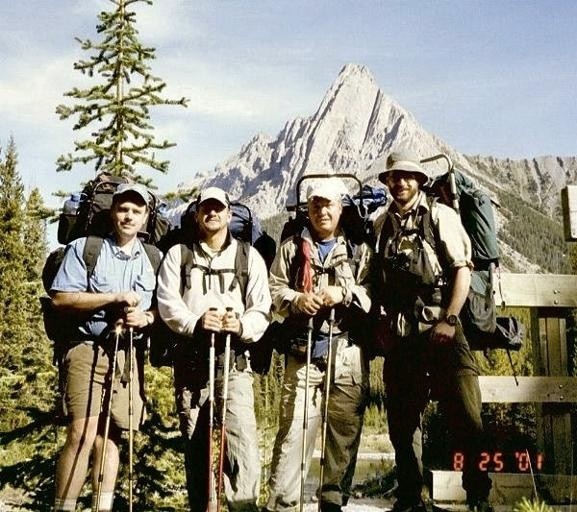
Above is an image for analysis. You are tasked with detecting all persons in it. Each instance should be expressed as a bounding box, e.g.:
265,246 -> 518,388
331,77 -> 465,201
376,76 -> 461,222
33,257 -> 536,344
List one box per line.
261,183 -> 374,512
154,186 -> 271,512
361,151 -> 492,512
50,183 -> 164,512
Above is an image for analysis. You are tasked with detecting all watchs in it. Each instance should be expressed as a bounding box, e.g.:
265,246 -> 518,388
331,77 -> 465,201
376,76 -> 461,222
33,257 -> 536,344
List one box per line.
443,313 -> 459,327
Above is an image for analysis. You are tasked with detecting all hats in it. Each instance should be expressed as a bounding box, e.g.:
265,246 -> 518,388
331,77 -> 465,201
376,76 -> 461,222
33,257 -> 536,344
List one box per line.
111,183 -> 151,209
306,179 -> 345,202
197,186 -> 229,207
378,154 -> 430,186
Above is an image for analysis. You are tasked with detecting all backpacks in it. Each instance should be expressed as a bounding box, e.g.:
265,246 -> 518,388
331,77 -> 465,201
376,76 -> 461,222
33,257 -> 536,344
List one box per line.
147,200 -> 274,375
278,175 -> 389,361
40,171 -> 174,339
421,156 -> 524,352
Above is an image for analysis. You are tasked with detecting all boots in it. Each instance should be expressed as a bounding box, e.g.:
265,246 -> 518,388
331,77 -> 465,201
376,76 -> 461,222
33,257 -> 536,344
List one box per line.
384,496 -> 427,512
465,489 -> 494,512
321,503 -> 344,512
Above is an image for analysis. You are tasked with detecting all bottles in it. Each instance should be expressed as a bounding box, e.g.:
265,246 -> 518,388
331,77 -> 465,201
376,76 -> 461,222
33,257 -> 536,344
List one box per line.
64,194 -> 80,214
